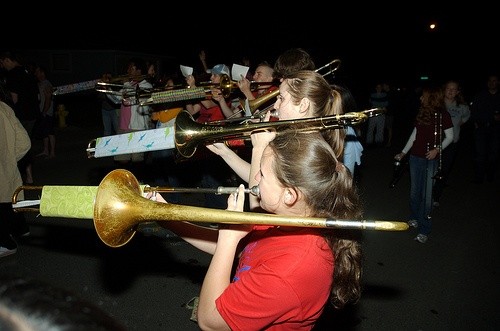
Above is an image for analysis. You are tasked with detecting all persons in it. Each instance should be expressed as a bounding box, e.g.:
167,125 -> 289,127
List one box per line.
383,83 -> 395,147
470,75 -> 500,143
366,84 -> 387,144
144,134 -> 363,331
432,81 -> 470,207
394,86 -> 454,244
100,48 -> 362,217
0,54 -> 68,259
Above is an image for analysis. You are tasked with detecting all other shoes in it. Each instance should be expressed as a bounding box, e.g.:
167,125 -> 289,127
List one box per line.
0,247 -> 17,258
407,218 -> 419,228
416,234 -> 430,244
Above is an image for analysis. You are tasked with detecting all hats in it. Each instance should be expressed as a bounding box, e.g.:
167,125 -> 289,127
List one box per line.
206,64 -> 230,78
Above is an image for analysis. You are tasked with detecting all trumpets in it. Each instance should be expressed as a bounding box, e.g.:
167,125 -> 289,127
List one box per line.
221,72 -> 281,98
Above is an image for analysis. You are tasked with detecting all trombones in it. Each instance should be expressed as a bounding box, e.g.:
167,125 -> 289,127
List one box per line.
11,167 -> 412,248
50,73 -> 154,97
87,108 -> 386,163
96,82 -> 225,108
216,57 -> 341,134
427,108 -> 444,181
